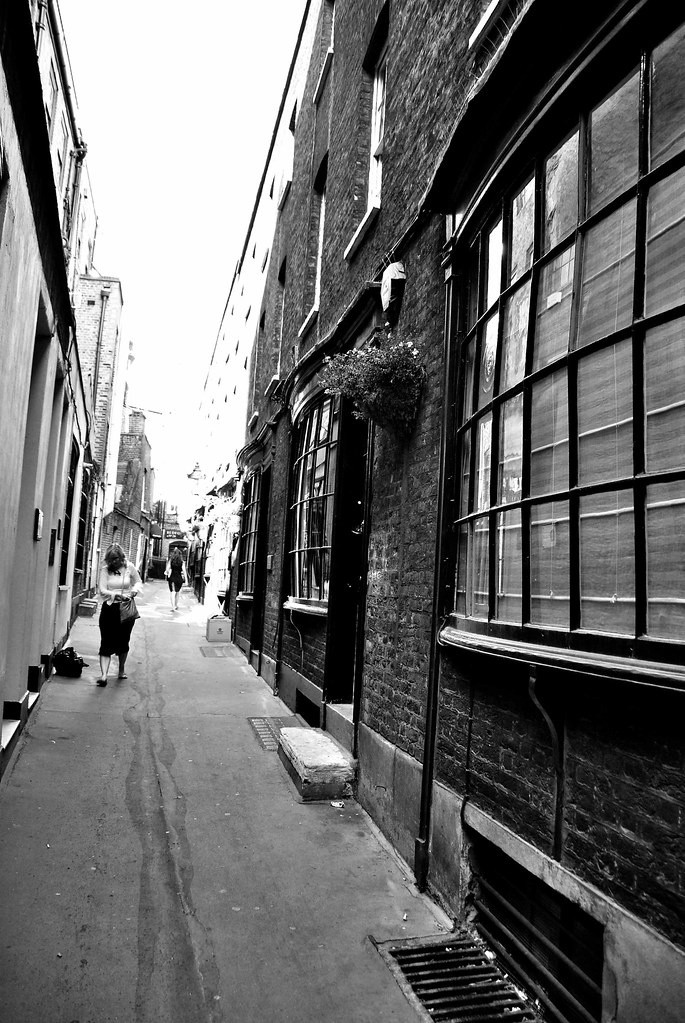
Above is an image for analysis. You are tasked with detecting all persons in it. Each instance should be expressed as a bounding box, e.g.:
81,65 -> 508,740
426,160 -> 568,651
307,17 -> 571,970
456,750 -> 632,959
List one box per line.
96,543 -> 143,686
163,546 -> 188,612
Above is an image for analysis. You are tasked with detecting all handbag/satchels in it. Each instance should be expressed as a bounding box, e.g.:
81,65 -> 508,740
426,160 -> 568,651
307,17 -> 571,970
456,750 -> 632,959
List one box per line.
120,598 -> 140,623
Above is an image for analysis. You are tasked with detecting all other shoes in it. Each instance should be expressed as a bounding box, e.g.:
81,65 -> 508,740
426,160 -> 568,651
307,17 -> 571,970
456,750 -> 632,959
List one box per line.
97,678 -> 108,686
118,673 -> 127,679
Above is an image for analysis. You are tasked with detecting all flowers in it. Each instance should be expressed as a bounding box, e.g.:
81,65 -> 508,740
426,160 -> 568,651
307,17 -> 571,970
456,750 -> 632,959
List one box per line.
316,343 -> 426,442
